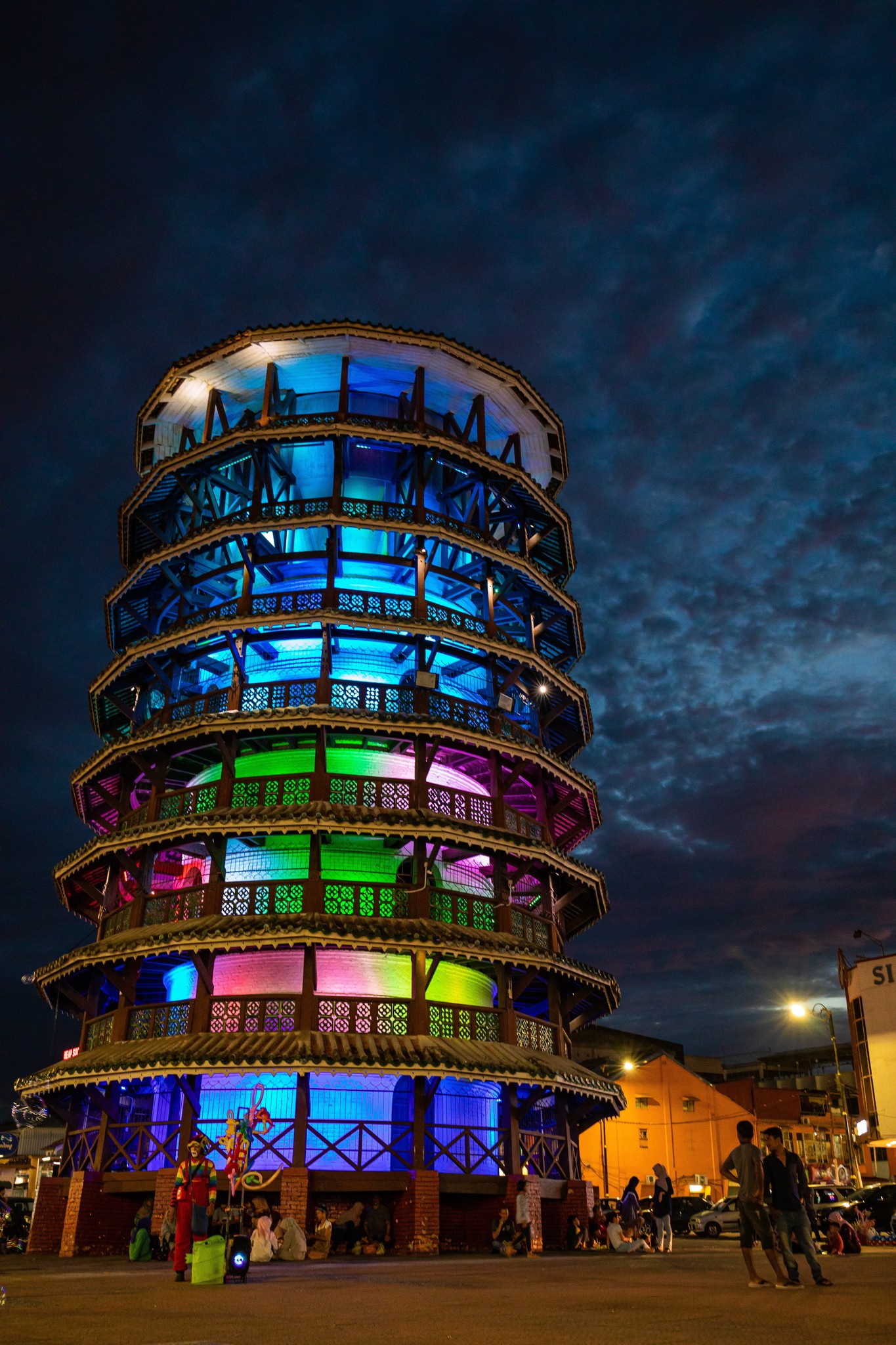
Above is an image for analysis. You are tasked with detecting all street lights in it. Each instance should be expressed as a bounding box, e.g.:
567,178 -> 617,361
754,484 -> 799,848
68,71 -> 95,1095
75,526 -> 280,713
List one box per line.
600,1055 -> 636,1199
787,1001 -> 857,1187
34,1157 -> 51,1195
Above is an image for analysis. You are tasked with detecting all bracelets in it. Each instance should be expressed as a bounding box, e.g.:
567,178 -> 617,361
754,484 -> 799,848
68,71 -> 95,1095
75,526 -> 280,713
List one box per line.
608,1248 -> 610,1251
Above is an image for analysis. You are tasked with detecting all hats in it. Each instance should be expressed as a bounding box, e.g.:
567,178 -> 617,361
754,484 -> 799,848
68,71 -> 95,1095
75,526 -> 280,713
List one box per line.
318,1204 -> 328,1215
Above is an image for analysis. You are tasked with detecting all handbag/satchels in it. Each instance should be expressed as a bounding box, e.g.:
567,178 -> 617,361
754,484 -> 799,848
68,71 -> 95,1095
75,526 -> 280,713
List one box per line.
376,1242 -> 386,1256
191,1204 -> 209,1235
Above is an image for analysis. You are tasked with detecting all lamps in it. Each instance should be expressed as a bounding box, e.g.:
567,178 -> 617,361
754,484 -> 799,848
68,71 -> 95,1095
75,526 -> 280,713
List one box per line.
416,671 -> 436,689
492,692 -> 515,712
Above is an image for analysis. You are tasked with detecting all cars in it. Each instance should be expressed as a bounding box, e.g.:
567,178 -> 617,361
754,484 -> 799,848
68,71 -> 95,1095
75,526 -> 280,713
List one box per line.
3,1197 -> 35,1218
638,1185 -> 863,1238
816,1182 -> 896,1233
599,1198 -> 622,1214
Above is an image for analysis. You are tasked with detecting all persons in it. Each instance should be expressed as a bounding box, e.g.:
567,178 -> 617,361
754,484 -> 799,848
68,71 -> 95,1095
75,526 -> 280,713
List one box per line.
0,1187 -> 7,1214
269,1211 -> 284,1240
331,1202 -> 366,1258
210,1197 -> 240,1239
278,1217 -> 307,1260
590,1205 -> 614,1249
820,1211 -> 861,1254
700,1192 -> 713,1205
305,1205 -> 332,1260
565,1214 -> 591,1251
649,1164 -> 674,1253
127,1198 -> 175,1262
620,1176 -> 643,1227
170,1134 -> 217,1281
762,1127 -> 834,1286
364,1193 -> 395,1254
506,1179 -> 539,1258
250,1216 -> 278,1262
490,1207 -> 522,1253
719,1120 -> 806,1290
606,1212 -> 656,1254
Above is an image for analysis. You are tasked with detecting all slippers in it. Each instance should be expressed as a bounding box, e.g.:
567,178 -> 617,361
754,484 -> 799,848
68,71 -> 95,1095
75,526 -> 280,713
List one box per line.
775,1281 -> 804,1290
748,1279 -> 773,1288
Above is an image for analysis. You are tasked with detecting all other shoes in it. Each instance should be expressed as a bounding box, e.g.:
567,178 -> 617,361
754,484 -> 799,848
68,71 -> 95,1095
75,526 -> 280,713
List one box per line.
790,1277 -> 801,1285
645,1247 -> 656,1254
656,1247 -> 663,1253
665,1249 -> 671,1253
527,1251 -> 538,1258
175,1270 -> 184,1281
815,1278 -> 833,1286
